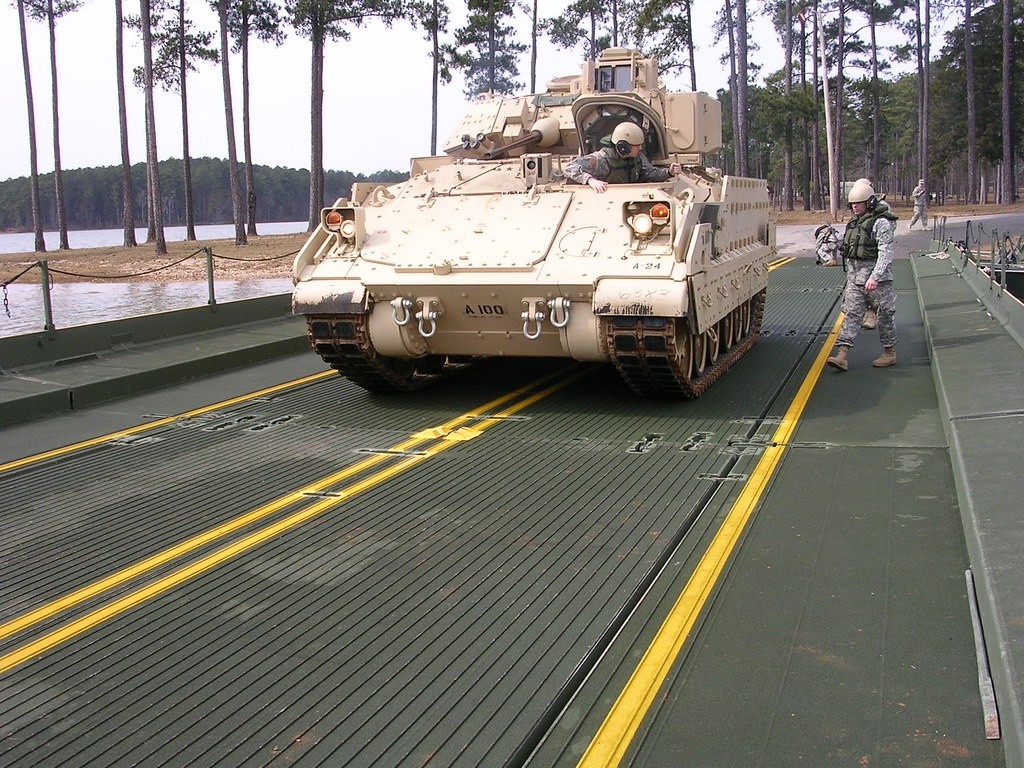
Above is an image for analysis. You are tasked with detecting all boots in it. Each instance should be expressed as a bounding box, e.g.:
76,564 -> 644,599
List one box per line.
861,308 -> 876,329
828,346 -> 848,371
873,347 -> 897,368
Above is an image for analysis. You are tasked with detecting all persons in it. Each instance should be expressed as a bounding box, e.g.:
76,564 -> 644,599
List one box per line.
827,183 -> 898,371
563,122 -> 681,194
908,179 -> 931,231
854,178 -> 897,328
813,225 -> 843,266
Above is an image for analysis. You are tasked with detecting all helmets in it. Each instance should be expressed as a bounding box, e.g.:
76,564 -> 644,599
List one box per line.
611,122 -> 644,145
848,183 -> 874,203
854,179 -> 871,186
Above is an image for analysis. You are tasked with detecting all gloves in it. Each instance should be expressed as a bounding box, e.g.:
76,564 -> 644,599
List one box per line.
816,260 -> 821,265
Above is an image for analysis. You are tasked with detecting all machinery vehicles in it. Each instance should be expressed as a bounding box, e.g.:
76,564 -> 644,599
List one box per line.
291,47 -> 777,405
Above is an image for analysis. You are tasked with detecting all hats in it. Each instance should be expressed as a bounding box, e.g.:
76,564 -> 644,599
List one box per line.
919,179 -> 925,184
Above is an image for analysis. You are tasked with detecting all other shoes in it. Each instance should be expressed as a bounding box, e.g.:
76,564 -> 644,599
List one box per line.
923,226 -> 931,231
908,225 -> 912,230
824,259 -> 837,266
